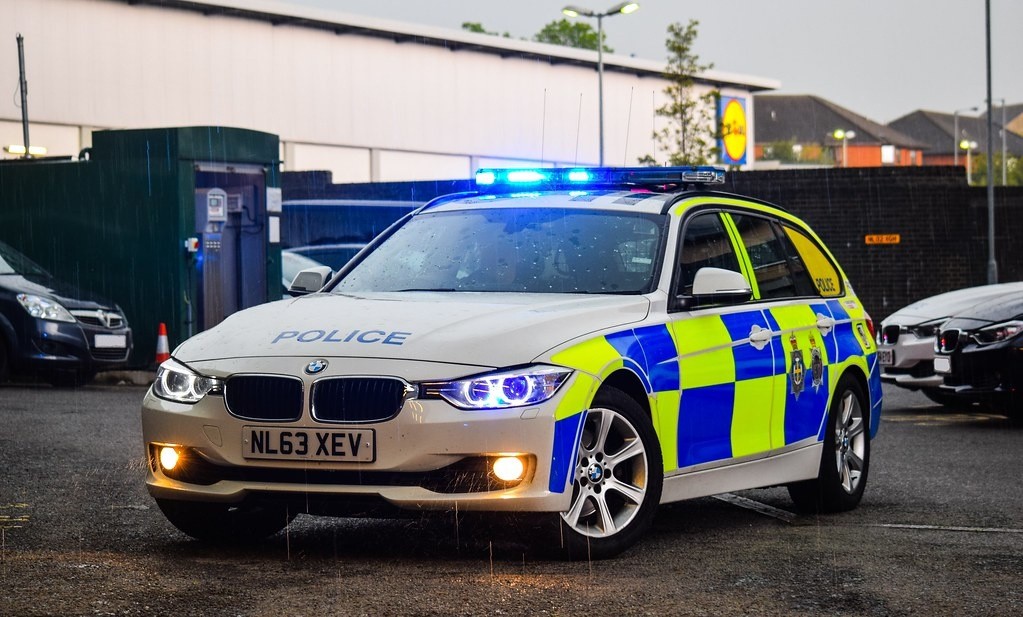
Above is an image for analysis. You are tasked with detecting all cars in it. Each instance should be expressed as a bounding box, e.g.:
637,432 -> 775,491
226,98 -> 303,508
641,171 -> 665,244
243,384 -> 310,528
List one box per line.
876,282 -> 1023,405
281,252 -> 339,301
0,241 -> 134,390
932,289 -> 1023,419
141,163 -> 886,559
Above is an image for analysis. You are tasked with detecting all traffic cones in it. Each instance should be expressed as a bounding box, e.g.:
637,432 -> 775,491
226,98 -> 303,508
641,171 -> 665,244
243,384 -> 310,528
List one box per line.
148,322 -> 170,371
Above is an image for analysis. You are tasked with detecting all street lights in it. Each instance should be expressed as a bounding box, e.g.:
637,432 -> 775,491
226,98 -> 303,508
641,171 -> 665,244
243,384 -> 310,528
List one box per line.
833,129 -> 857,167
560,2 -> 642,169
985,98 -> 1009,186
954,107 -> 979,168
960,139 -> 979,187
